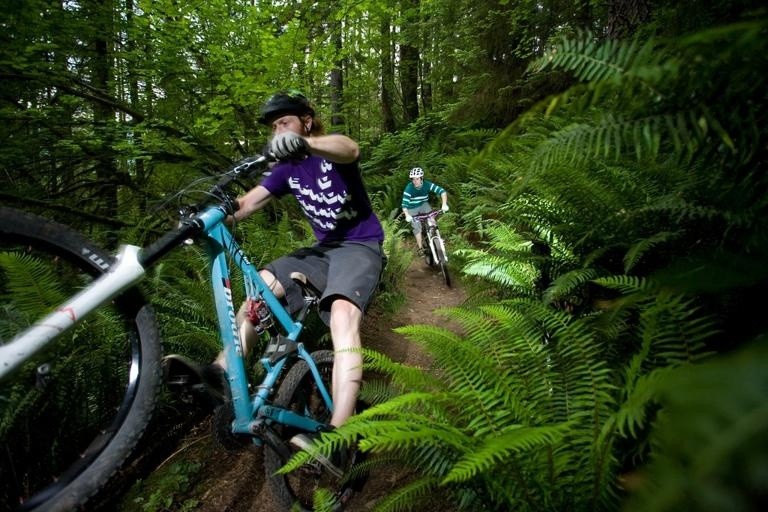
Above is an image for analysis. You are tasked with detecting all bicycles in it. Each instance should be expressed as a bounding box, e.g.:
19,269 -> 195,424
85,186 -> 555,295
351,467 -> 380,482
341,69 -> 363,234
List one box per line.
412,208 -> 451,290
0,143 -> 373,511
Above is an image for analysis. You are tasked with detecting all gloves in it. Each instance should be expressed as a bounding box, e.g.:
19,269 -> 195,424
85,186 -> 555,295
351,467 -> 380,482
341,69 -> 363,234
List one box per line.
442,202 -> 449,214
264,132 -> 310,164
406,215 -> 413,222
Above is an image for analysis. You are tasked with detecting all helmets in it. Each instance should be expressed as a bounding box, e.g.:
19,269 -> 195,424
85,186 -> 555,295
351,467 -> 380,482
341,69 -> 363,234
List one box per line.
409,167 -> 424,179
258,90 -> 315,126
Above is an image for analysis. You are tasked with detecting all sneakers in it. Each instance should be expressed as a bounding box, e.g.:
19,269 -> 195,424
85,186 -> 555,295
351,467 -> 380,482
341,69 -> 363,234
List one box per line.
161,355 -> 231,406
417,249 -> 425,258
290,426 -> 350,479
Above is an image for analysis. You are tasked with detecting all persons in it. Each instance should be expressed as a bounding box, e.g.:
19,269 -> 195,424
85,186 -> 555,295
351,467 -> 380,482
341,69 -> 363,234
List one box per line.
164,91 -> 388,479
400,167 -> 449,259
